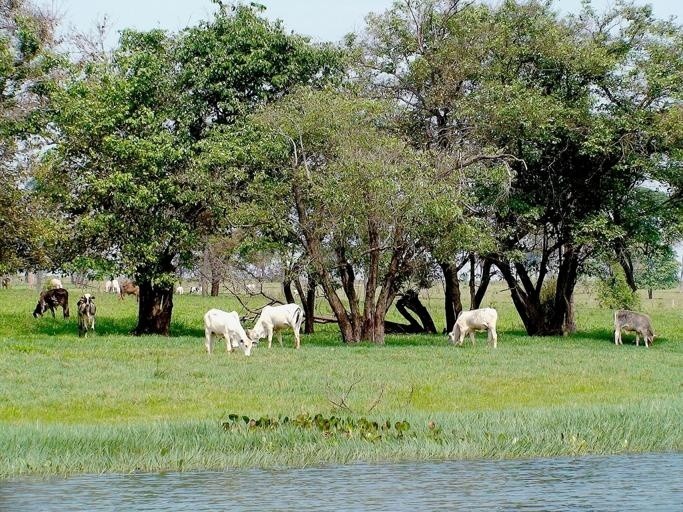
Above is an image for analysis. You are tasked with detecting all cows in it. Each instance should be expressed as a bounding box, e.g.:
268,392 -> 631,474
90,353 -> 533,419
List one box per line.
175,286 -> 200,295
448,307 -> 497,349
105,279 -> 138,302
77,293 -> 95,340
614,308 -> 654,348
32,288 -> 68,320
1,278 -> 9,290
246,303 -> 303,349
203,308 -> 257,357
49,278 -> 62,291
